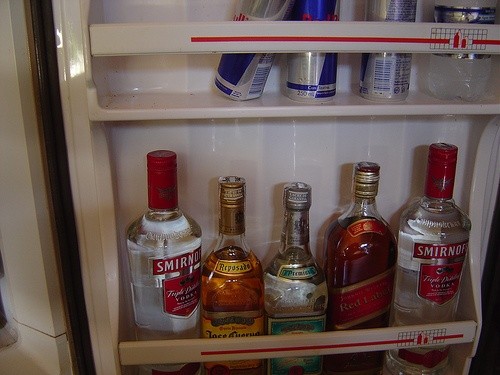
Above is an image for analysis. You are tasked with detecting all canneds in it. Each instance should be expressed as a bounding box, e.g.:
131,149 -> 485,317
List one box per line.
359,0 -> 419,102
214,0 -> 293,101
283,0 -> 342,103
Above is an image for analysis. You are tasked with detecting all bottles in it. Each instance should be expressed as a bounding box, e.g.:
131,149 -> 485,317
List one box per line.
324,162 -> 396,375
128,150 -> 201,373
200,176 -> 265,368
428,0 -> 497,102
386,143 -> 471,375
262,182 -> 326,375
